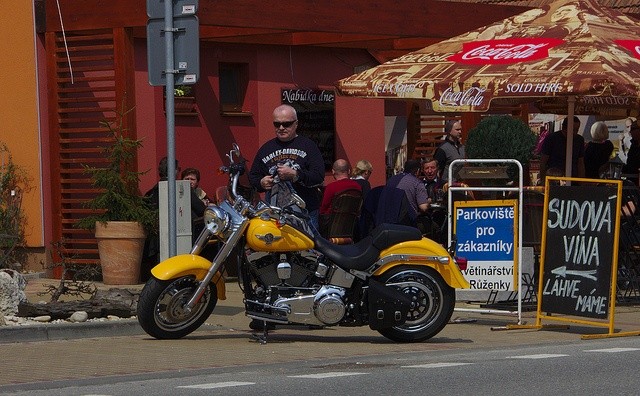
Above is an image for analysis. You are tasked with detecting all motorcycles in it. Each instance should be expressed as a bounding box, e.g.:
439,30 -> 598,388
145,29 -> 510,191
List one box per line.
138,142 -> 471,344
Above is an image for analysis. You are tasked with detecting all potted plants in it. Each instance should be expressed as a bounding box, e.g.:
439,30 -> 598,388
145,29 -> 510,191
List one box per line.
164,84 -> 196,113
73,87 -> 158,284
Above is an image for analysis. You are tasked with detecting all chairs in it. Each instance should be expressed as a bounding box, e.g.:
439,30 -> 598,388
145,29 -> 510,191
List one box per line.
442,181 -> 477,202
189,214 -> 221,252
507,184 -> 546,198
324,192 -> 363,246
367,184 -> 413,226
216,184 -> 232,205
609,176 -> 639,295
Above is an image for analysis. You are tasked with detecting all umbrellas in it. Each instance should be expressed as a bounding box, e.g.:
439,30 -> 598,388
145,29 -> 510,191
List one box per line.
334,0 -> 640,185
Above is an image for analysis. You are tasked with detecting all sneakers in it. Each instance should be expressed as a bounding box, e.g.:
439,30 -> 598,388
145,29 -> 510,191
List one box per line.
250,320 -> 264,329
267,322 -> 275,329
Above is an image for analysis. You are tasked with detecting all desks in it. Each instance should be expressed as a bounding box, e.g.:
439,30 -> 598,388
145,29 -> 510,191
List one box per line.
418,201 -> 446,235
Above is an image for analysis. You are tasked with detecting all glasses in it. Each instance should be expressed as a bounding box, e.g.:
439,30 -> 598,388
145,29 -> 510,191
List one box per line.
175,166 -> 181,172
274,120 -> 296,127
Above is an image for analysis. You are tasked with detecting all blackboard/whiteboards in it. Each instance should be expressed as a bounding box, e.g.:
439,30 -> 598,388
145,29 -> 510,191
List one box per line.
538,176 -> 623,328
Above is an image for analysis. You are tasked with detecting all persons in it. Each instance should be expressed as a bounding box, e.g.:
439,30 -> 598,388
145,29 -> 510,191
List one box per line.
418,156 -> 449,205
528,2 -> 592,42
376,158 -> 433,225
620,119 -> 640,175
350,159 -> 373,201
433,118 -> 466,186
246,103 -> 326,332
446,4 -> 552,41
539,115 -> 584,189
318,157 -> 362,234
181,168 -> 218,210
142,156 -> 208,282
583,120 -> 615,178
